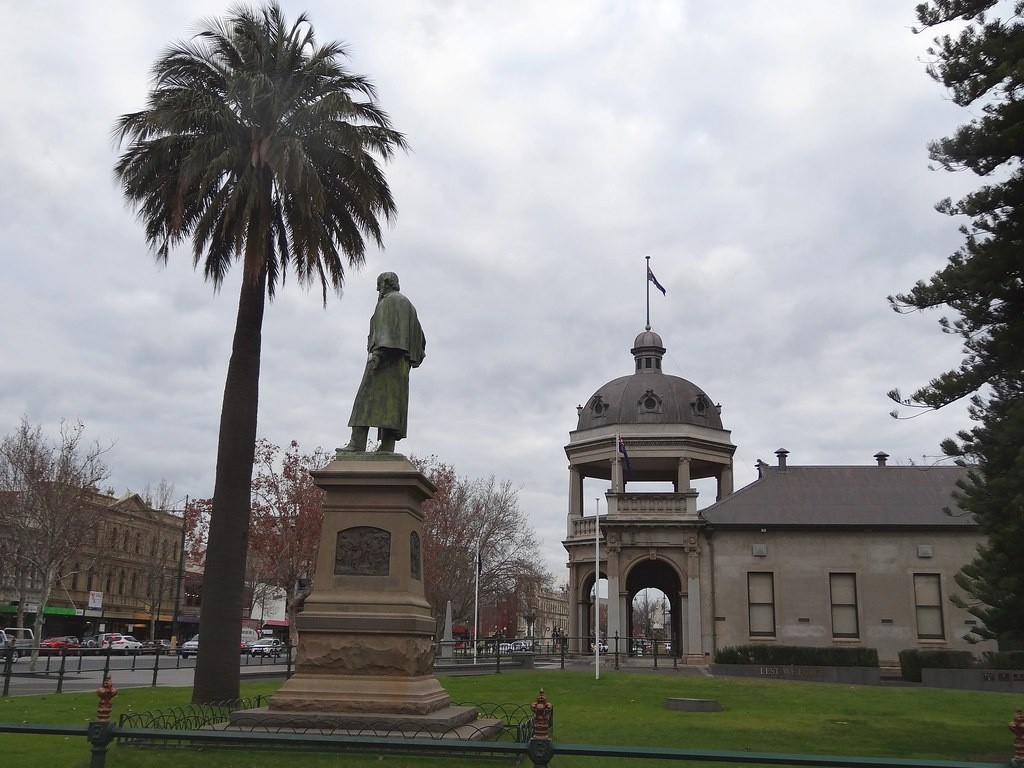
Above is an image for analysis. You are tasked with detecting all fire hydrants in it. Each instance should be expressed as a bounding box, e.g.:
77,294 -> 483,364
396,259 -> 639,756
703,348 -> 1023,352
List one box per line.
527,688 -> 557,768
88,672 -> 119,768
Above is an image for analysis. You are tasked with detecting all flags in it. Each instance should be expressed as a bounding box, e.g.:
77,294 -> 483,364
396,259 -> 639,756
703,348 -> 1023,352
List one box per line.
648,268 -> 666,296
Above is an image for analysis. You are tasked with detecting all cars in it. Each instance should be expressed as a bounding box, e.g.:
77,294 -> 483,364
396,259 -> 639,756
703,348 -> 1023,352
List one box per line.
634,642 -> 671,655
0,627 -> 182,663
240,637 -> 288,658
183,634 -> 200,658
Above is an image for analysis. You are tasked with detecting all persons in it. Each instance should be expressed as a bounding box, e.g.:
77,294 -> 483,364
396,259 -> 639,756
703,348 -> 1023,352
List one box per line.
334,272 -> 426,453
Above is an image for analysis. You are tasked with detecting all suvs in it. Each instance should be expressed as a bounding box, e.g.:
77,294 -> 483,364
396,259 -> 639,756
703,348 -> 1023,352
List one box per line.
593,643 -> 607,653
496,642 -> 531,653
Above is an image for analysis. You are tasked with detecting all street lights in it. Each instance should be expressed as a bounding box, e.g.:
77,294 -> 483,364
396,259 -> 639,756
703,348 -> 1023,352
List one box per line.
593,497 -> 599,678
473,521 -> 492,663
125,494 -> 212,641
